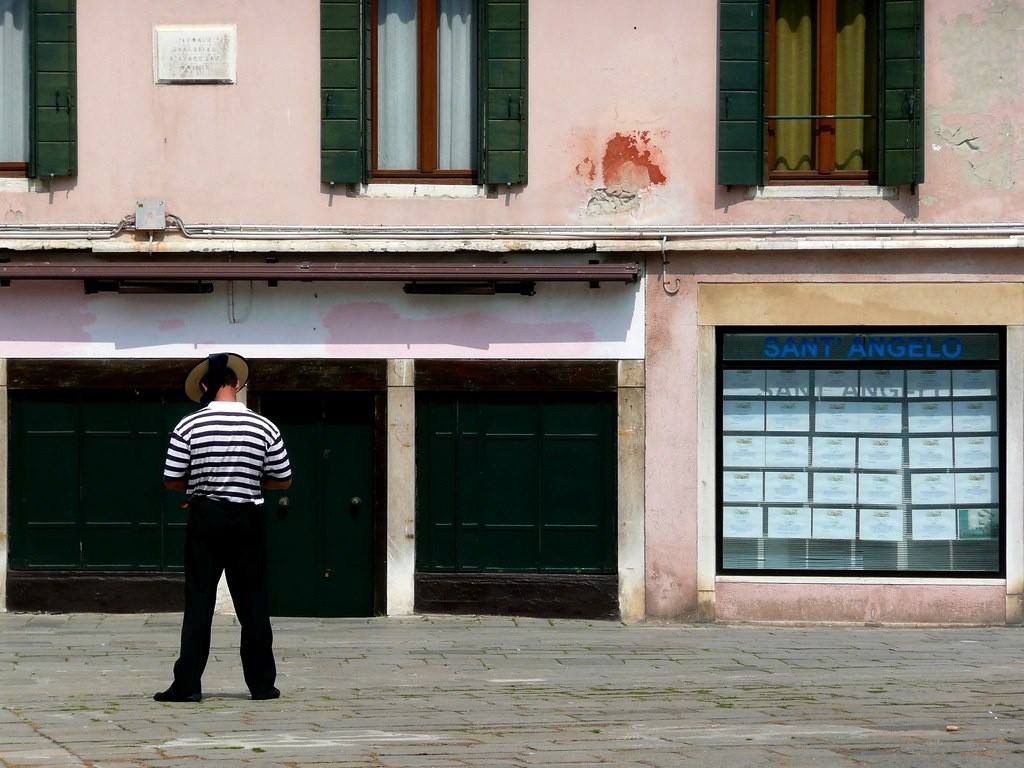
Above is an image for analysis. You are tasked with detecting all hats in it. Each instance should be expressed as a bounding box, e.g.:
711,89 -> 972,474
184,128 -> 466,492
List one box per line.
184,352 -> 249,403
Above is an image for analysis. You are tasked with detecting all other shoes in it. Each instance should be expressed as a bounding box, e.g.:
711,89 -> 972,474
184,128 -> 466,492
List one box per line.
252,686 -> 280,700
154,681 -> 202,702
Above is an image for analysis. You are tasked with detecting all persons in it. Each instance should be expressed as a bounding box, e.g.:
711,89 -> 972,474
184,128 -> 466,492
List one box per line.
153,353 -> 292,702
